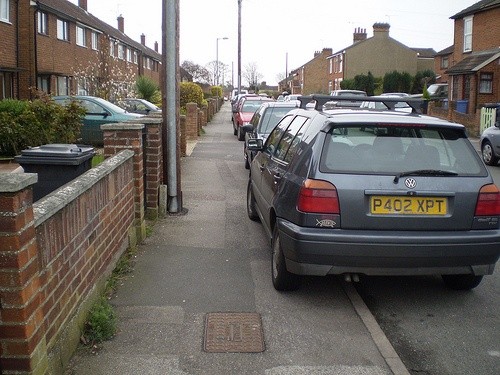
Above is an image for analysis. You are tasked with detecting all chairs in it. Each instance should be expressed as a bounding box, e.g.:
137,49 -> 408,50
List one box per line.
325,140 -> 440,173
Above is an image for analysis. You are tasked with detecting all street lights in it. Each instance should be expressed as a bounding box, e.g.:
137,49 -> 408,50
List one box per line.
216,37 -> 229,113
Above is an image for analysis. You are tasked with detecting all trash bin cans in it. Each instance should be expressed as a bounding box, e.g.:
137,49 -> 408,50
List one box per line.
13,144 -> 96,203
456,100 -> 469,115
444,99 -> 448,110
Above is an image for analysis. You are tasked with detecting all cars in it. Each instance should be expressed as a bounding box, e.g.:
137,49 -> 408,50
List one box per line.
248,93 -> 499,293
480,102 -> 500,166
109,97 -> 164,114
230,84 -> 450,140
31,96 -> 142,143
244,102 -> 326,170
